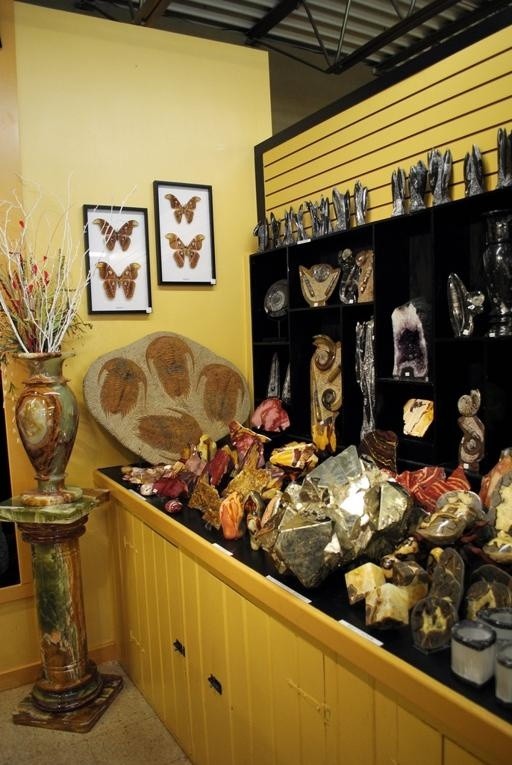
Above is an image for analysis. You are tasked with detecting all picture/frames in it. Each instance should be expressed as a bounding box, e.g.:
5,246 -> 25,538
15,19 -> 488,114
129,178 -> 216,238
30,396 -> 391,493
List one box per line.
154,180 -> 216,286
84,204 -> 153,314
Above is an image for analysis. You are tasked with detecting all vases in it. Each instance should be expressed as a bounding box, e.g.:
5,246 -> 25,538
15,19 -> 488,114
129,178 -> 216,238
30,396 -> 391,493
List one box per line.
12,350 -> 79,507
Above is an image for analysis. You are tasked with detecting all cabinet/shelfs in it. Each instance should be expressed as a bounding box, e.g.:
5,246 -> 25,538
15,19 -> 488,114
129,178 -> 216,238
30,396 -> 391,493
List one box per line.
90,187 -> 512,764
